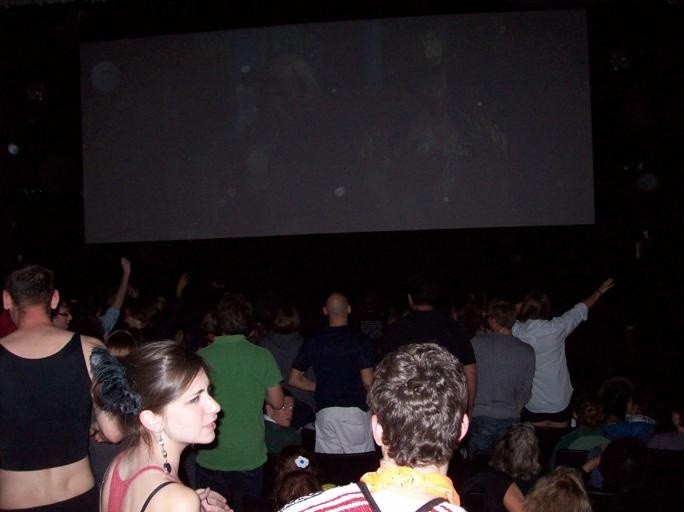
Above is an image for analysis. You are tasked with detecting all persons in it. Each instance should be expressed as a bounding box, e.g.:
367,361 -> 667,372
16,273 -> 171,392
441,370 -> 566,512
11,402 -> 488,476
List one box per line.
0,266 -> 126,512
52,258 -> 476,482
90,340 -> 235,511
446,278 -> 684,511
275,344 -> 469,512
272,470 -> 336,512
195,292 -> 285,512
